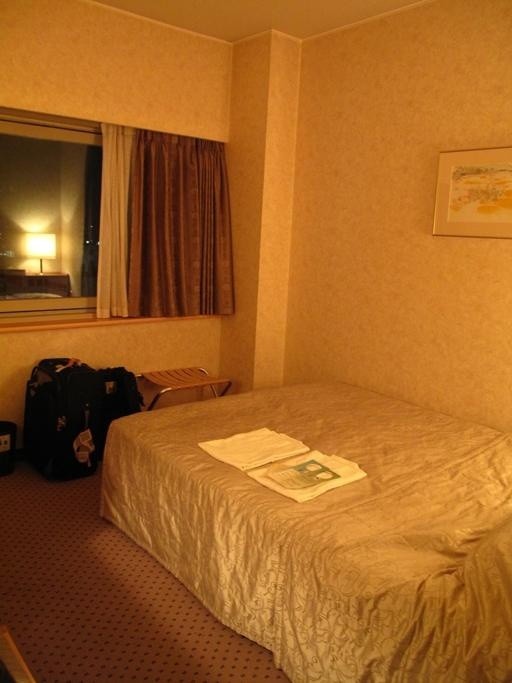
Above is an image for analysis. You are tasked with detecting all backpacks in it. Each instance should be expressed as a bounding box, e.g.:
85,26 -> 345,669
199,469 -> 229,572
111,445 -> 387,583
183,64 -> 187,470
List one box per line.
99,364 -> 146,461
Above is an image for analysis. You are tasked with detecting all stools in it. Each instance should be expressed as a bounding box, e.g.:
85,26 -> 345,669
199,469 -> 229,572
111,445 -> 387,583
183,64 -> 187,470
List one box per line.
132,366 -> 232,407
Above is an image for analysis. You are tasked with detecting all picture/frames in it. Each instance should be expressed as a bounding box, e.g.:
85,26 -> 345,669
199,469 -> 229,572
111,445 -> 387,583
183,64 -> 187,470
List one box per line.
431,148 -> 512,241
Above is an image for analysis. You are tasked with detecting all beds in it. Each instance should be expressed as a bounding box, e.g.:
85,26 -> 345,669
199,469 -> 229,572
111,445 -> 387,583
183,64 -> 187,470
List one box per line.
105,382 -> 509,677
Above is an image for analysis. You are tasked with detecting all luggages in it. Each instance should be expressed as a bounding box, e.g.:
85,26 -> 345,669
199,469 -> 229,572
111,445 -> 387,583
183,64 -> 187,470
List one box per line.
20,357 -> 102,483
